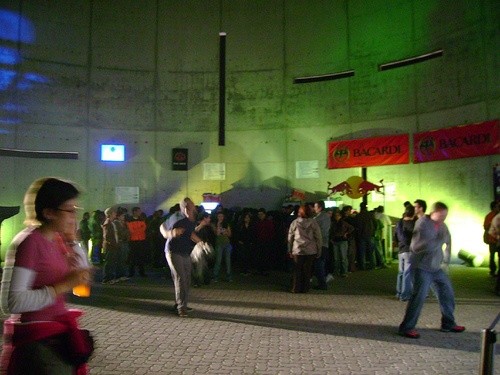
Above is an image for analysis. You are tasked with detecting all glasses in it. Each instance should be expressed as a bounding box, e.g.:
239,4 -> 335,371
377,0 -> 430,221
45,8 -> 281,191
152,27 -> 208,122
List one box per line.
56,207 -> 76,213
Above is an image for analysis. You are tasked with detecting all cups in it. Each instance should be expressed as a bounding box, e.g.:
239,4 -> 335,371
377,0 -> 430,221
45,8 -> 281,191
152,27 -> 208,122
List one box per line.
73,265 -> 101,297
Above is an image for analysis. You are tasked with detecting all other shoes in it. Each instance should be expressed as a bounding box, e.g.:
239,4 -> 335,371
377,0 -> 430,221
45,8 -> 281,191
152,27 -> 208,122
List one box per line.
185,306 -> 194,312
177,310 -> 188,317
440,325 -> 465,332
383,263 -> 392,268
140,274 -> 147,277
289,288 -> 296,293
397,330 -> 421,338
325,275 -> 334,282
103,277 -> 131,284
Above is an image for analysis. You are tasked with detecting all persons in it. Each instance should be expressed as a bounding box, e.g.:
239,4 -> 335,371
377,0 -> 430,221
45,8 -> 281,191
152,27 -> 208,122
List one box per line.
394,199 -> 429,302
281,200 -> 392,294
8,179 -> 94,375
484,199 -> 500,296
78,204 -> 181,284
190,205 -> 286,287
160,198 -> 214,317
399,202 -> 466,339
